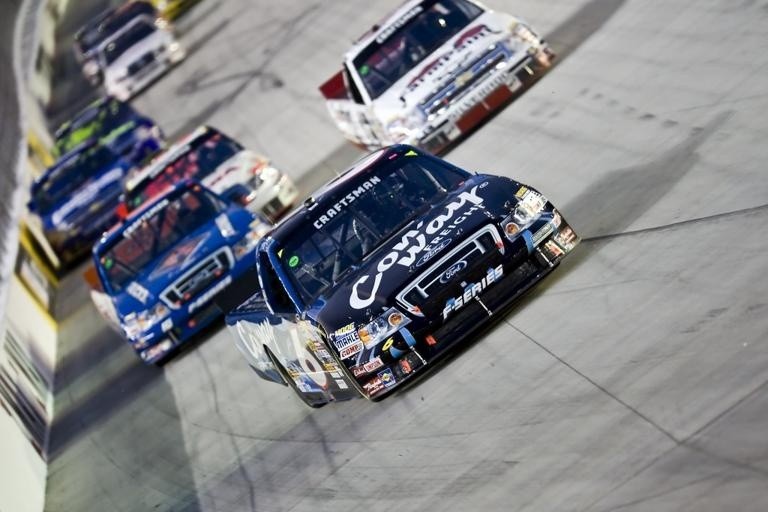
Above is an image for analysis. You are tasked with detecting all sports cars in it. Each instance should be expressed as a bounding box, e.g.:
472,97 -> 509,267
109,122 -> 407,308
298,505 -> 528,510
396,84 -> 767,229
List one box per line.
317,0 -> 557,158
90,181 -> 274,368
223,144 -> 575,403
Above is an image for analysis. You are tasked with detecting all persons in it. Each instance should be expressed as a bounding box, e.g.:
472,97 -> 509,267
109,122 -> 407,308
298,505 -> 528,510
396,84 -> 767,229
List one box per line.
370,176 -> 423,226
177,206 -> 203,231
